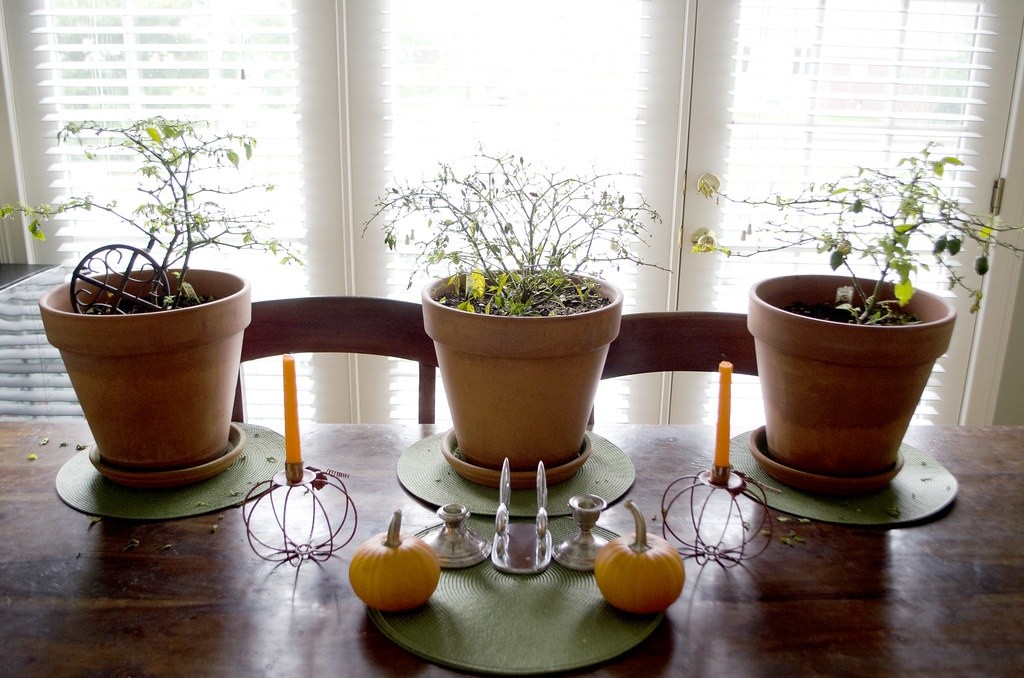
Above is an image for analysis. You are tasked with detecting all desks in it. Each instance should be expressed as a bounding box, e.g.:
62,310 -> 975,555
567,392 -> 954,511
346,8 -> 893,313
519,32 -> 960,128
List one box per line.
0,422 -> 1024,678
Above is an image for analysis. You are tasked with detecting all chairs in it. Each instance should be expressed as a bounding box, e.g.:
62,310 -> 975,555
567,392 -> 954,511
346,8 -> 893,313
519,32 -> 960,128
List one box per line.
587,312 -> 760,426
231,297 -> 440,424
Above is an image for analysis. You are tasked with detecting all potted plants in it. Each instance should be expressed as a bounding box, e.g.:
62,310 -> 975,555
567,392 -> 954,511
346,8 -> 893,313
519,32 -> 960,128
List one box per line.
689,140 -> 1024,496
0,115 -> 307,488
359,143 -> 675,483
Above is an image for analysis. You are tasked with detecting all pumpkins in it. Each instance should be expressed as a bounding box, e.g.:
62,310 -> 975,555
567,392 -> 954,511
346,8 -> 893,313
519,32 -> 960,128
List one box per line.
349,509 -> 440,610
594,499 -> 684,615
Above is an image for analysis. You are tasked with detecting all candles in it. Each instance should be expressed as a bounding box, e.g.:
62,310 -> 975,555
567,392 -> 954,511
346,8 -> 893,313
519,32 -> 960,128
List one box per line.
712,352 -> 734,468
281,350 -> 306,464
